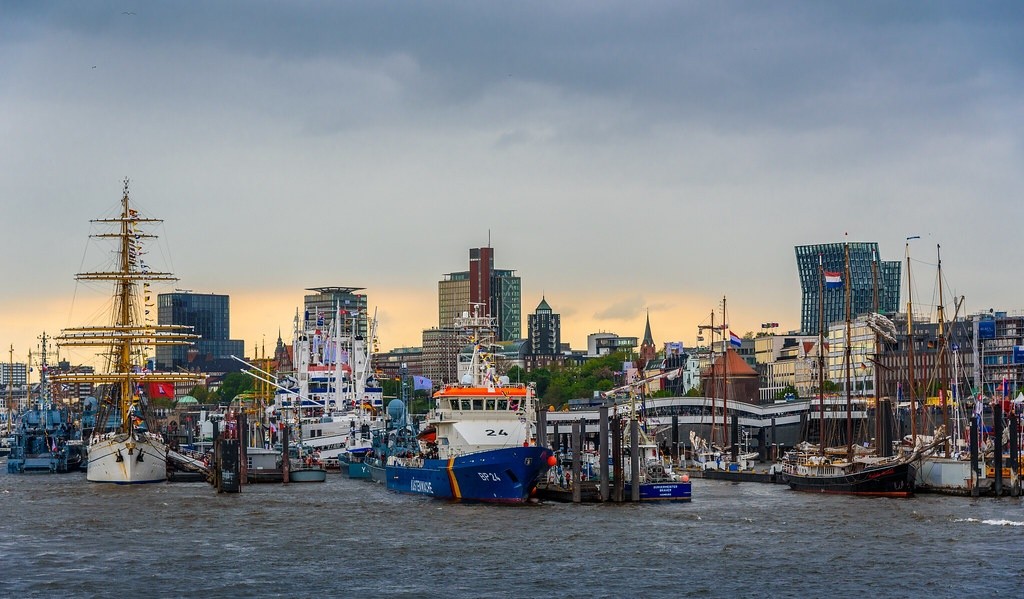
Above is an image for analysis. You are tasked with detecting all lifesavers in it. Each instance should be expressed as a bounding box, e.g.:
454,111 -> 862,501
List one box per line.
170,440 -> 178,451
656,466 -> 664,474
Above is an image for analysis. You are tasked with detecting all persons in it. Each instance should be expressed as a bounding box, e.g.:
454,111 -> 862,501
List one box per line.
397,450 -> 415,458
381,454 -> 386,466
497,402 -> 507,410
510,401 -> 519,410
155,431 -> 179,452
365,450 -> 378,463
488,402 -> 494,410
417,446 -> 439,459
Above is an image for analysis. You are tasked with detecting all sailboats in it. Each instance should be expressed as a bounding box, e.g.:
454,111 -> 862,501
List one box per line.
6,170 -> 760,511
778,236 -> 1024,499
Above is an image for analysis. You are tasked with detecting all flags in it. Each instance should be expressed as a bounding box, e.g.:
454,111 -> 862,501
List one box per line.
730,332 -> 741,347
132,368 -> 148,428
414,376 -> 432,390
150,381 -> 175,397
364,402 -> 376,411
825,271 -> 843,288
762,323 -> 778,328
